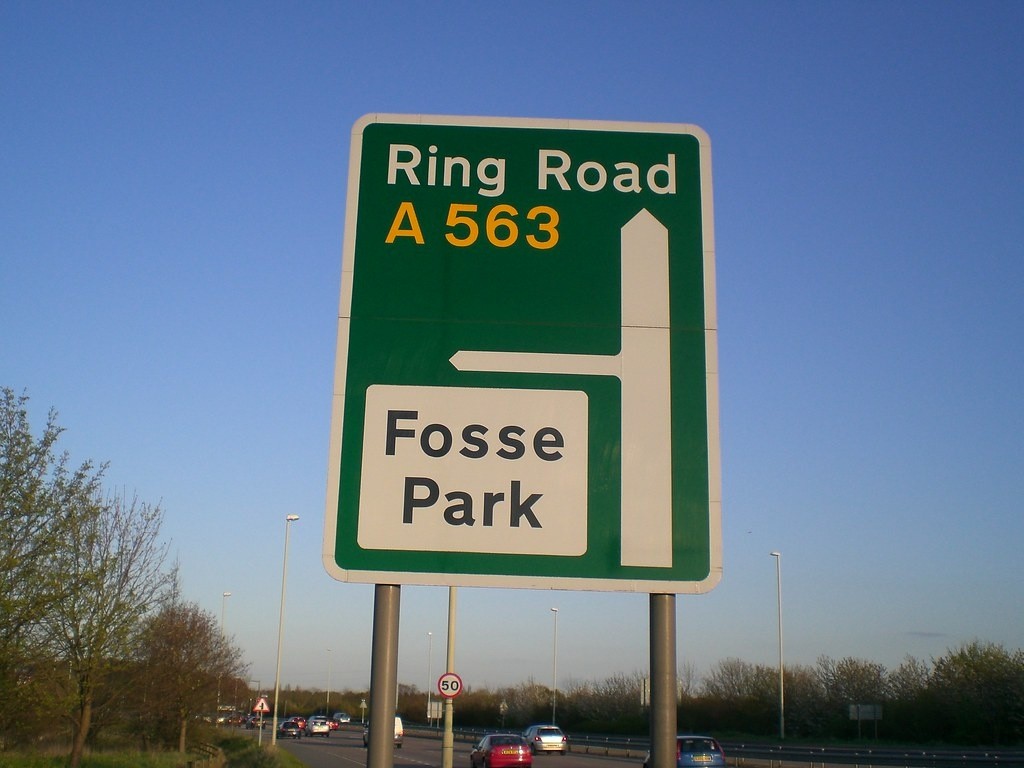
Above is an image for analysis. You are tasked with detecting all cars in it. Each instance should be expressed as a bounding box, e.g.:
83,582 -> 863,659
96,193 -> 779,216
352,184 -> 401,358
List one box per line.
303,716 -> 339,738
469,734 -> 533,768
213,711 -> 267,729
333,713 -> 350,723
275,722 -> 301,740
523,725 -> 567,756
641,736 -> 726,768
362,717 -> 404,749
288,717 -> 306,730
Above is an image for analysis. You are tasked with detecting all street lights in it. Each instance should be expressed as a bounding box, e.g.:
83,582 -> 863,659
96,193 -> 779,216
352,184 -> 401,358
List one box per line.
271,514 -> 300,746
550,607 -> 559,726
214,592 -> 233,733
769,552 -> 785,743
428,632 -> 433,731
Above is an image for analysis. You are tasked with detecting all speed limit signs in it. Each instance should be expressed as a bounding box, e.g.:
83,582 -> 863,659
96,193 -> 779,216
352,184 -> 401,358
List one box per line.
437,673 -> 464,700
